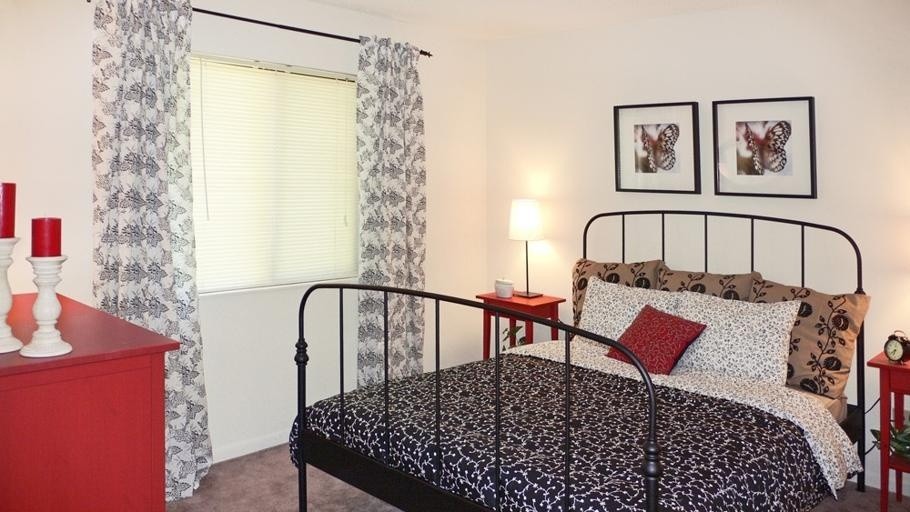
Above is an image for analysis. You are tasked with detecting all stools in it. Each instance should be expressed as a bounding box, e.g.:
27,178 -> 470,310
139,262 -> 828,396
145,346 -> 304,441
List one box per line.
476,290 -> 566,360
865,352 -> 909,512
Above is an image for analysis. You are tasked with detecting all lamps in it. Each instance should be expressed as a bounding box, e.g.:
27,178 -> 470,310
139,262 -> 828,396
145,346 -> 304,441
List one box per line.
507,197 -> 548,299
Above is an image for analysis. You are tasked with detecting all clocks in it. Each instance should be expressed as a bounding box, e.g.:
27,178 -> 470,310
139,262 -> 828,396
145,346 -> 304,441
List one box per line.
883,326 -> 910,367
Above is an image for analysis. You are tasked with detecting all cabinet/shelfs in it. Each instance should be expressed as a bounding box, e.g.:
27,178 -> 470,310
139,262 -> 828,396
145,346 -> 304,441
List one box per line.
0,290 -> 181,512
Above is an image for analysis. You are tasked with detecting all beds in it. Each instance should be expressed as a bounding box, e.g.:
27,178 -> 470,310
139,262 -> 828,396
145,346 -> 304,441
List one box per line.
288,207 -> 867,512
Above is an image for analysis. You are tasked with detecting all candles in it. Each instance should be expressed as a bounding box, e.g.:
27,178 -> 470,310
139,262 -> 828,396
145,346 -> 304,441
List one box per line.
0,182 -> 15,239
32,217 -> 62,257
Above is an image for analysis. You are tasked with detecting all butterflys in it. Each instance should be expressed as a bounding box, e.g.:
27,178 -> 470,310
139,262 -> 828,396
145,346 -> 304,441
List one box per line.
743,121 -> 792,175
641,123 -> 680,175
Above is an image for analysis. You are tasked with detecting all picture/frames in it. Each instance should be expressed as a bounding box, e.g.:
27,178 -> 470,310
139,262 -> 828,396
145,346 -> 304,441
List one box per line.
710,94 -> 819,202
613,101 -> 703,196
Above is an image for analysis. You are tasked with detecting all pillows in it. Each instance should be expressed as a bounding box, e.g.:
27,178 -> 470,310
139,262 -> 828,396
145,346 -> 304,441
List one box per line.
751,278 -> 873,402
683,288 -> 804,388
571,275 -> 683,352
656,261 -> 751,306
606,304 -> 709,377
570,257 -> 665,340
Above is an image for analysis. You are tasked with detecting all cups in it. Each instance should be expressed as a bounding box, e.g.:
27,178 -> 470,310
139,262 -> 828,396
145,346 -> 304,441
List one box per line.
496,279 -> 514,299
